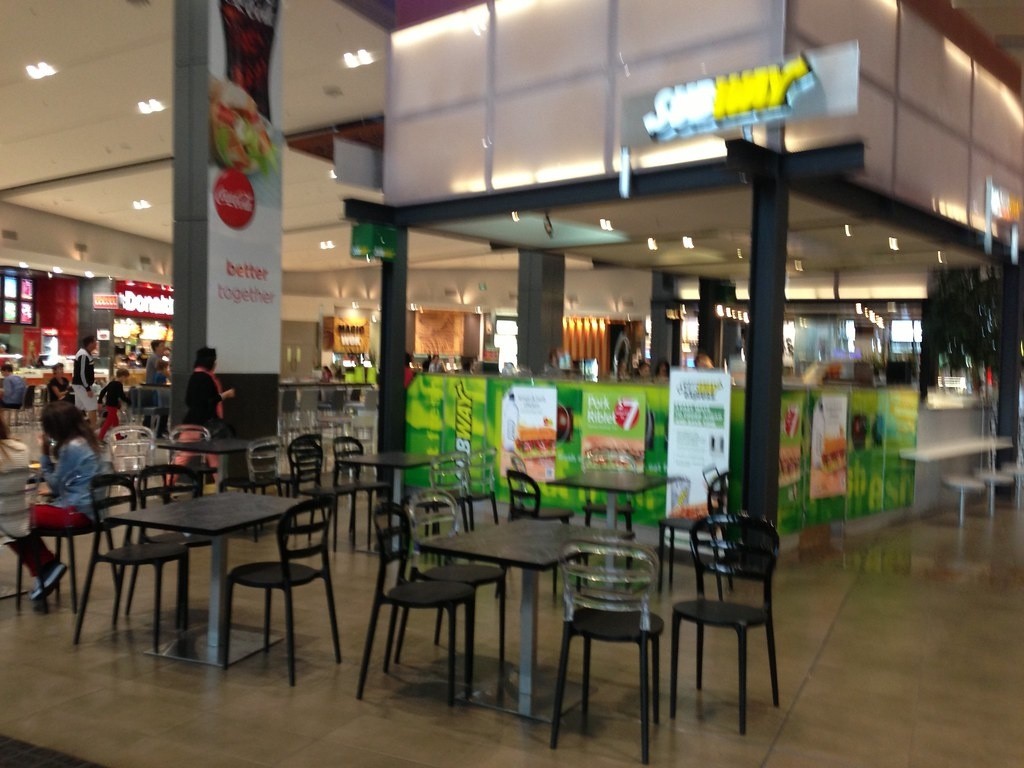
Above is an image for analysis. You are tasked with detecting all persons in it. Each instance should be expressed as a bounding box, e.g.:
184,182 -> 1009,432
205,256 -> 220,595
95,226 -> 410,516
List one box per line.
322,366 -> 332,382
166,346 -> 235,497
46,336 -> 171,445
0,364 -> 26,409
632,360 -> 670,385
423,354 -> 447,372
9,403 -> 104,614
0,419 -> 29,538
404,350 -> 417,390
694,354 -> 714,369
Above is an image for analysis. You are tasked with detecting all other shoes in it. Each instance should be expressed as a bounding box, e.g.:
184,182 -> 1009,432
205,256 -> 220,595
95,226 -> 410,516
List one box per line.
33,598 -> 49,612
97,439 -> 105,448
120,435 -> 128,440
30,561 -> 67,600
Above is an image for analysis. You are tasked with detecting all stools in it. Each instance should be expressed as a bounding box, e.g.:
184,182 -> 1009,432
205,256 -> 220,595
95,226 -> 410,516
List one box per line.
973,469 -> 1013,518
940,474 -> 985,527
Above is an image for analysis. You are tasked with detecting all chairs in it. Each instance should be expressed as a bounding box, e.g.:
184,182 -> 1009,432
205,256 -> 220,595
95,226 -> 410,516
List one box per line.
668,513 -> 783,736
426,451 -> 499,530
221,434 -> 391,554
279,379 -> 379,431
395,490 -> 507,701
111,464 -> 214,630
72,472 -> 191,653
539,537 -> 661,764
355,501 -> 472,711
222,497 -> 344,688
657,473 -> 735,601
0,384 -> 212,612
494,469 -> 574,607
580,447 -> 638,572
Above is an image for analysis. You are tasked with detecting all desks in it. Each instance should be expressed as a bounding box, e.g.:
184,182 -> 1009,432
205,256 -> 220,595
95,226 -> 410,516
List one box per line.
544,468 -> 676,591
335,451 -> 438,553
157,440 -> 285,481
104,492 -> 313,669
418,519 -> 634,724
